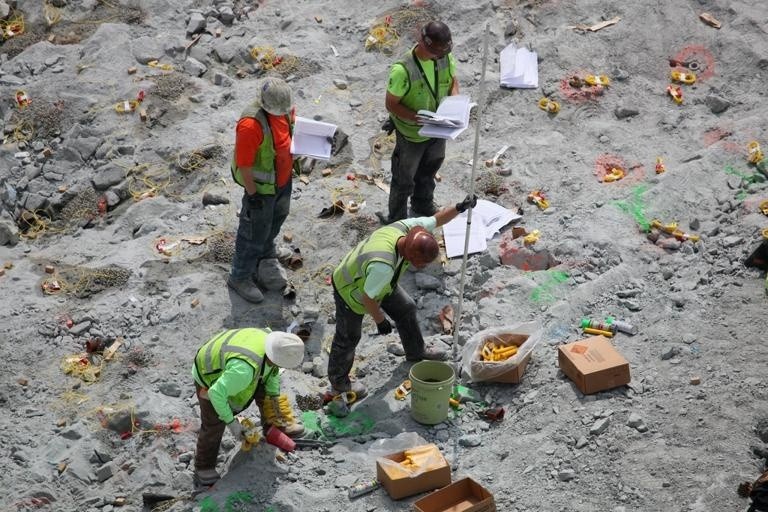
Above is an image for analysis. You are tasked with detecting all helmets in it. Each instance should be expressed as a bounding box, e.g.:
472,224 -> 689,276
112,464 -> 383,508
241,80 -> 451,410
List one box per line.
421,20 -> 454,56
256,76 -> 295,116
407,226 -> 438,271
226,275 -> 264,304
265,331 -> 305,367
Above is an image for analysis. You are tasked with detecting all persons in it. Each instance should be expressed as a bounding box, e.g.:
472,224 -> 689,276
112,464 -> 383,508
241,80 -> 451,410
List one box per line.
327,195 -> 477,400
189,325 -> 306,487
384,19 -> 460,223
230,76 -> 333,305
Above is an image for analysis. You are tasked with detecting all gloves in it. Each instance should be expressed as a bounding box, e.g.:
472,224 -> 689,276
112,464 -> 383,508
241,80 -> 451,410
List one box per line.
227,418 -> 250,441
377,318 -> 395,335
248,191 -> 267,211
456,194 -> 479,214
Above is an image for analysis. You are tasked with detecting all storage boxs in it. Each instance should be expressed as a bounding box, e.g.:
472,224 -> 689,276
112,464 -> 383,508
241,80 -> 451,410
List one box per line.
470,333 -> 630,395
376,443 -> 497,512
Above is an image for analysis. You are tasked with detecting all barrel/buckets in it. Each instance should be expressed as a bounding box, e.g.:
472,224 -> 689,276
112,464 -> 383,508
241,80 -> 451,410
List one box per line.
409,361 -> 455,424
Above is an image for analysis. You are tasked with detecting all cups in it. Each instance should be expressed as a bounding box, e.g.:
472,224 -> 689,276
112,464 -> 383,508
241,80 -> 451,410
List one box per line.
266,424 -> 297,452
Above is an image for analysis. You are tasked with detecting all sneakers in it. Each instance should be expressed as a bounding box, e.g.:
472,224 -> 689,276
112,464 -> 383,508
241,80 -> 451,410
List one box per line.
194,465 -> 221,486
329,382 -> 369,399
262,421 -> 307,438
406,347 -> 449,361
260,247 -> 294,260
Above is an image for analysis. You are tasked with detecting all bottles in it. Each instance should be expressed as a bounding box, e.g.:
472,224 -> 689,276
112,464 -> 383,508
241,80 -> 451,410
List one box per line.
608,319 -> 638,336
348,479 -> 381,498
580,318 -> 618,336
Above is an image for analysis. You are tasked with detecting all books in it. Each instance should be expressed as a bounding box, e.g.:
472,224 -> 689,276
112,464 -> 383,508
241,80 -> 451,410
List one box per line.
500,44 -> 538,89
289,116 -> 337,163
415,95 -> 476,140
